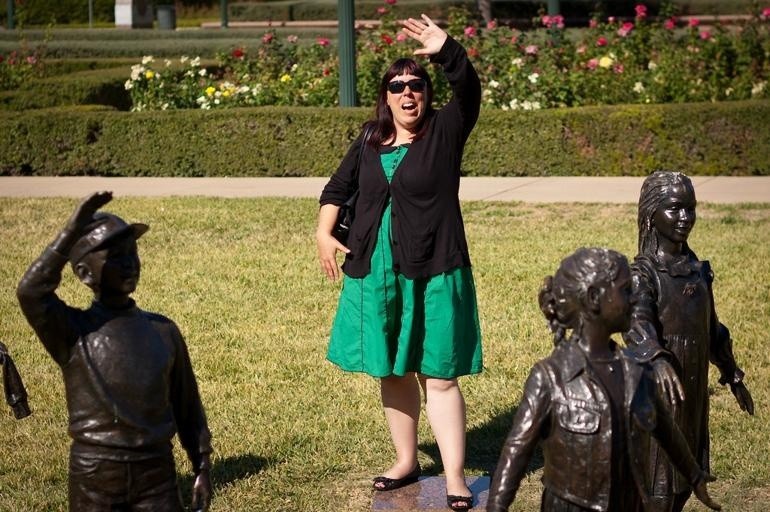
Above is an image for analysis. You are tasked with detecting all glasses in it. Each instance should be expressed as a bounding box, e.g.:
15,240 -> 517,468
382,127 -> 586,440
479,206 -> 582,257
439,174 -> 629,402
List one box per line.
386,78 -> 426,93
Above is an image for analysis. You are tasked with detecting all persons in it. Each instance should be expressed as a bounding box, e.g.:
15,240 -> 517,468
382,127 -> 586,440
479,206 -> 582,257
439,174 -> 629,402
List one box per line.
316,14 -> 483,511
17,191 -> 213,512
485,246 -> 720,512
619,171 -> 754,512
0,342 -> 31,420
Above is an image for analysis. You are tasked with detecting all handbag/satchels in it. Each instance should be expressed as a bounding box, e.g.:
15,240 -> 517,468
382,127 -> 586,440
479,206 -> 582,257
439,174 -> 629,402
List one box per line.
331,188 -> 361,247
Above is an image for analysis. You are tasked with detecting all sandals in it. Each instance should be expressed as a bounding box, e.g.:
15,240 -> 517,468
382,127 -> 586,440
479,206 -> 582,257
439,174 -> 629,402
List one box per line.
373,462 -> 421,491
445,486 -> 474,512
